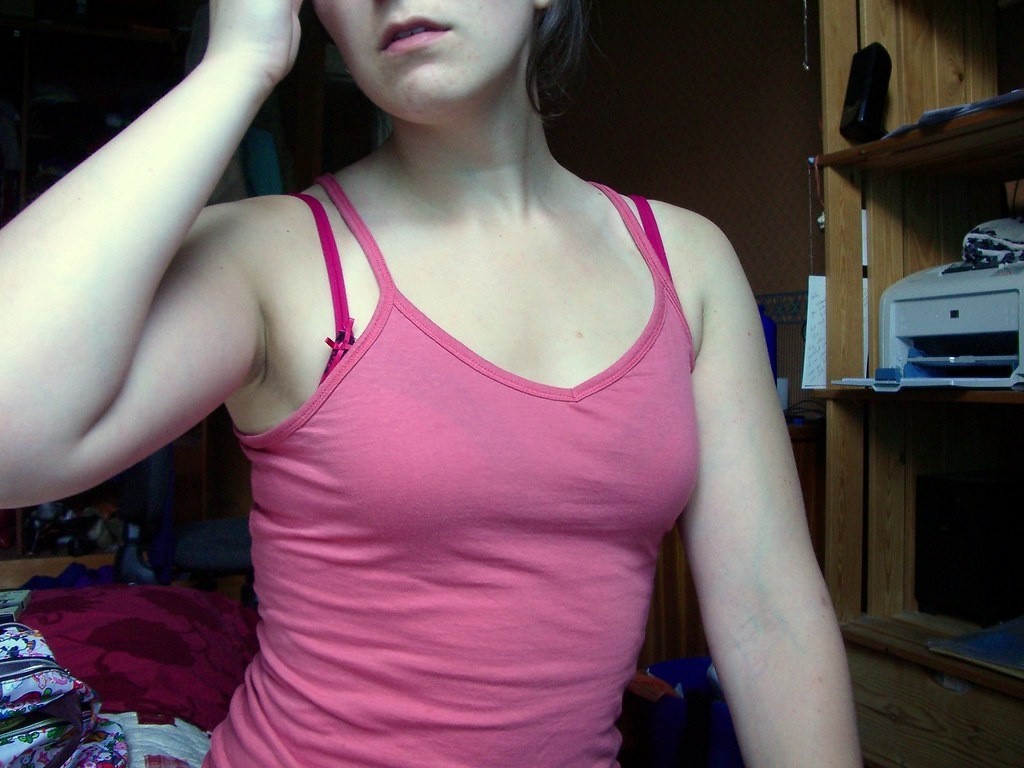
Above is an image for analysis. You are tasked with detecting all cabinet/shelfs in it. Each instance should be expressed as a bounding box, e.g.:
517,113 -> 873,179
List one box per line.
820,0 -> 1024,768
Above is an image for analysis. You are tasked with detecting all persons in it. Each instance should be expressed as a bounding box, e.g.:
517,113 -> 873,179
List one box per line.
1,1 -> 864,768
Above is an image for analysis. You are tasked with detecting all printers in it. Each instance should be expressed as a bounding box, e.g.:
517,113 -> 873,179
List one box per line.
875,259 -> 1024,391
